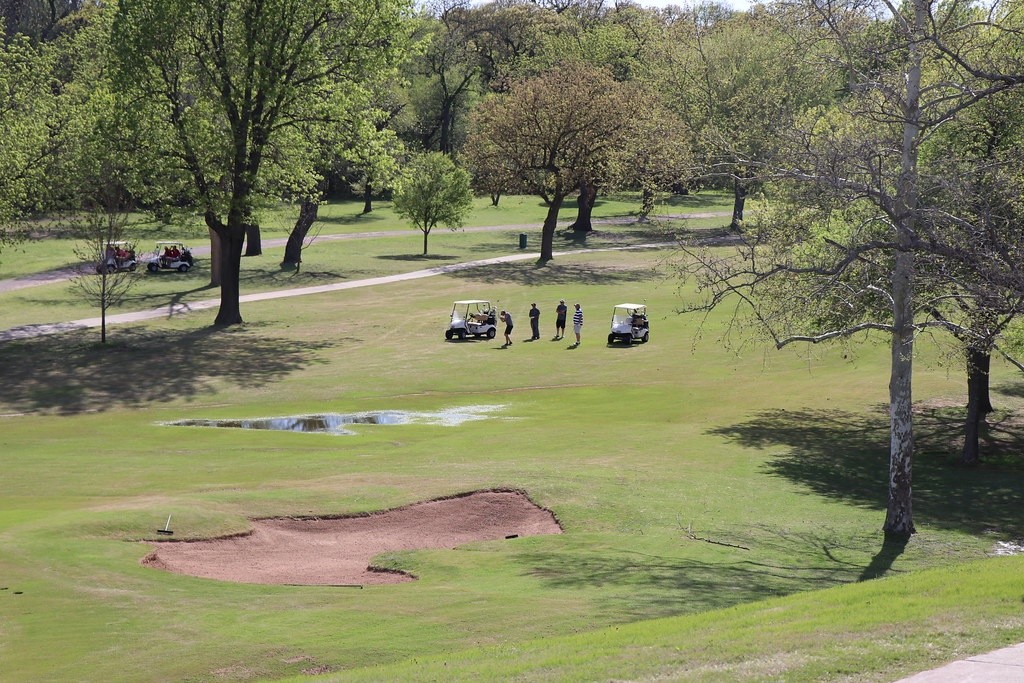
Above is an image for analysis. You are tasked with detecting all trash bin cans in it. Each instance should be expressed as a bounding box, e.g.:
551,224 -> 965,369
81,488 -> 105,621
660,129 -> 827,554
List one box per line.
520,232 -> 527,248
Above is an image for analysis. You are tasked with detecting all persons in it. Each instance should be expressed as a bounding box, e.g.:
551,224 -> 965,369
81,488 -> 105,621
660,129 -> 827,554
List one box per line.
630,309 -> 637,317
106,244 -> 126,257
161,246 -> 172,265
529,303 -> 540,340
572,304 -> 584,346
554,299 -> 567,338
500,310 -> 513,346
170,246 -> 179,258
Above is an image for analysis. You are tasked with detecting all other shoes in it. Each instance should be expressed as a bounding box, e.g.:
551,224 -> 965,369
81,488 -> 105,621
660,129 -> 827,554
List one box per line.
562,336 -> 564,338
505,344 -> 509,346
537,336 -> 540,339
554,335 -> 559,337
509,342 -> 512,345
574,341 -> 580,346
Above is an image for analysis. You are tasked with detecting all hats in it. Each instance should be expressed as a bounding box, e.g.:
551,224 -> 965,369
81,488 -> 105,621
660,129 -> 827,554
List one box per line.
531,303 -> 536,306
560,299 -> 564,303
574,303 -> 581,308
